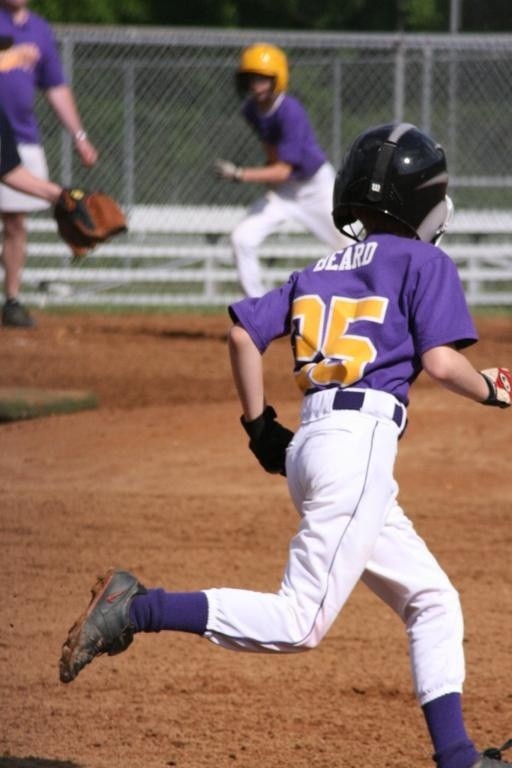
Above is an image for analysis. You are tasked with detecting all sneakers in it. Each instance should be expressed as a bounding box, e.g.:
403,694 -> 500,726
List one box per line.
3,298 -> 33,327
58,568 -> 148,684
472,739 -> 512,768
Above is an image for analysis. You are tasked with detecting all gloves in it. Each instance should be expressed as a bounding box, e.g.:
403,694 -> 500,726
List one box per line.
480,366 -> 512,408
211,159 -> 244,182
240,403 -> 296,478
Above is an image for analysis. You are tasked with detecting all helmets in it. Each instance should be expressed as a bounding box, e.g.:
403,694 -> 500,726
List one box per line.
332,123 -> 454,247
235,42 -> 290,96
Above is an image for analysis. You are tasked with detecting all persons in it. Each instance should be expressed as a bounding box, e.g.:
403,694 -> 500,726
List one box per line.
2,0 -> 98,329
209,41 -> 363,300
55,121 -> 511,767
0,109 -> 132,260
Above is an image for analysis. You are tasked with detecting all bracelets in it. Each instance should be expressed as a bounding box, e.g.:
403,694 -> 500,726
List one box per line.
73,131 -> 89,145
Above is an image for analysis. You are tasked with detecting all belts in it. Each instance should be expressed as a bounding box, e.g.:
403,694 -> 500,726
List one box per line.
335,390 -> 408,439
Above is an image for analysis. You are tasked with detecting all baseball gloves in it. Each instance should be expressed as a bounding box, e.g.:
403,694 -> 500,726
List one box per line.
54,187 -> 128,266
